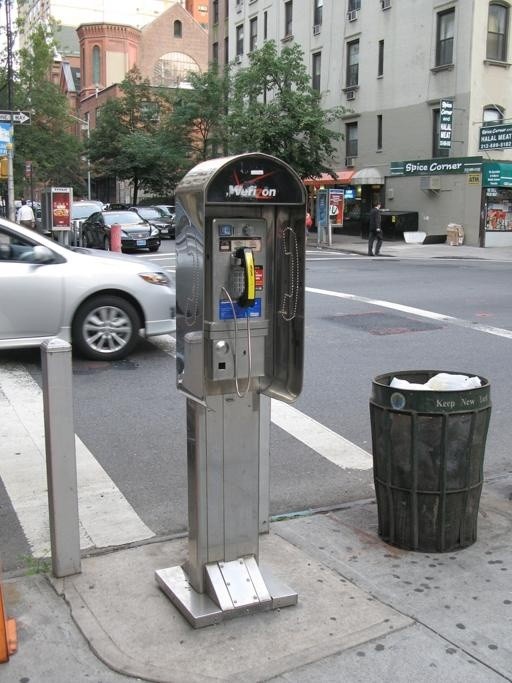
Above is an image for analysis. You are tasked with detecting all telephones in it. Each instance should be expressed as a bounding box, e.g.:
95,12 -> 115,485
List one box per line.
209,217 -> 266,322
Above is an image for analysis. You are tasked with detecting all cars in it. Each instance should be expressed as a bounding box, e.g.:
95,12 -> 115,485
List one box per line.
0,199 -> 177,251
0,218 -> 176,360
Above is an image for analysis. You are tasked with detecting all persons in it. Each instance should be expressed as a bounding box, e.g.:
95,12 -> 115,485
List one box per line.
16,199 -> 35,229
368,201 -> 383,256
305,212 -> 311,247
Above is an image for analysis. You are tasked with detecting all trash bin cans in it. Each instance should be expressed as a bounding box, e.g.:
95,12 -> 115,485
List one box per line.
368,369 -> 491,554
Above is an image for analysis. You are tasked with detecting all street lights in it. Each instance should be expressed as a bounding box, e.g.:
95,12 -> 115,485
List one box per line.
64,113 -> 91,200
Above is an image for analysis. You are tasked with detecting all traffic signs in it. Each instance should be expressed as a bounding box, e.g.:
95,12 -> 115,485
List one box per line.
0,110 -> 31,125
